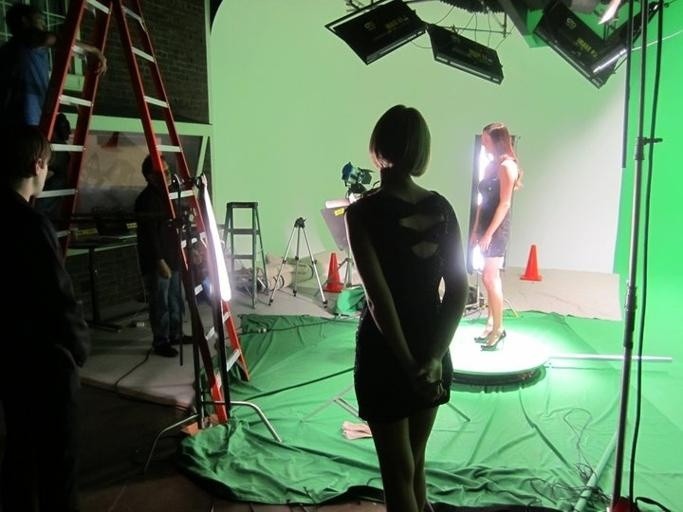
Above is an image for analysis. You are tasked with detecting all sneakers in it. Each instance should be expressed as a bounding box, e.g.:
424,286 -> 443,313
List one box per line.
156,335 -> 192,358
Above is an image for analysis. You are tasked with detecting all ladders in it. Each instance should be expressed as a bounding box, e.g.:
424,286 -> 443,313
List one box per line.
38,0 -> 270,424
222,201 -> 270,308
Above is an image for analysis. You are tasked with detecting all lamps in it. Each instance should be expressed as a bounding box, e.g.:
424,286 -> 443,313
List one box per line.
325,1 -> 620,92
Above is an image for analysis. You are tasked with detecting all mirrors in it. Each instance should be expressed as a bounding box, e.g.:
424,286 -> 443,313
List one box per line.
463,131 -> 517,274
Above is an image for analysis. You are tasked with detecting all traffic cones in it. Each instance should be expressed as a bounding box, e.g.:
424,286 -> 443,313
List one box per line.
323,251 -> 345,293
518,244 -> 542,281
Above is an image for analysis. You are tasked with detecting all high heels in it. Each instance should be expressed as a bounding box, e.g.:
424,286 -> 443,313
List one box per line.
475,329 -> 506,350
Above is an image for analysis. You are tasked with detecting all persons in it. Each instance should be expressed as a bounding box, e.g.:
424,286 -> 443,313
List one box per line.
0,124 -> 89,510
467,122 -> 522,352
0,0 -> 108,130
133,153 -> 193,356
33,113 -> 73,223
342,105 -> 467,510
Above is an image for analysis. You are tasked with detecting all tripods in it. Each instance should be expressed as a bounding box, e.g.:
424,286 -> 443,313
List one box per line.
268,217 -> 328,309
144,220 -> 281,473
314,249 -> 354,298
